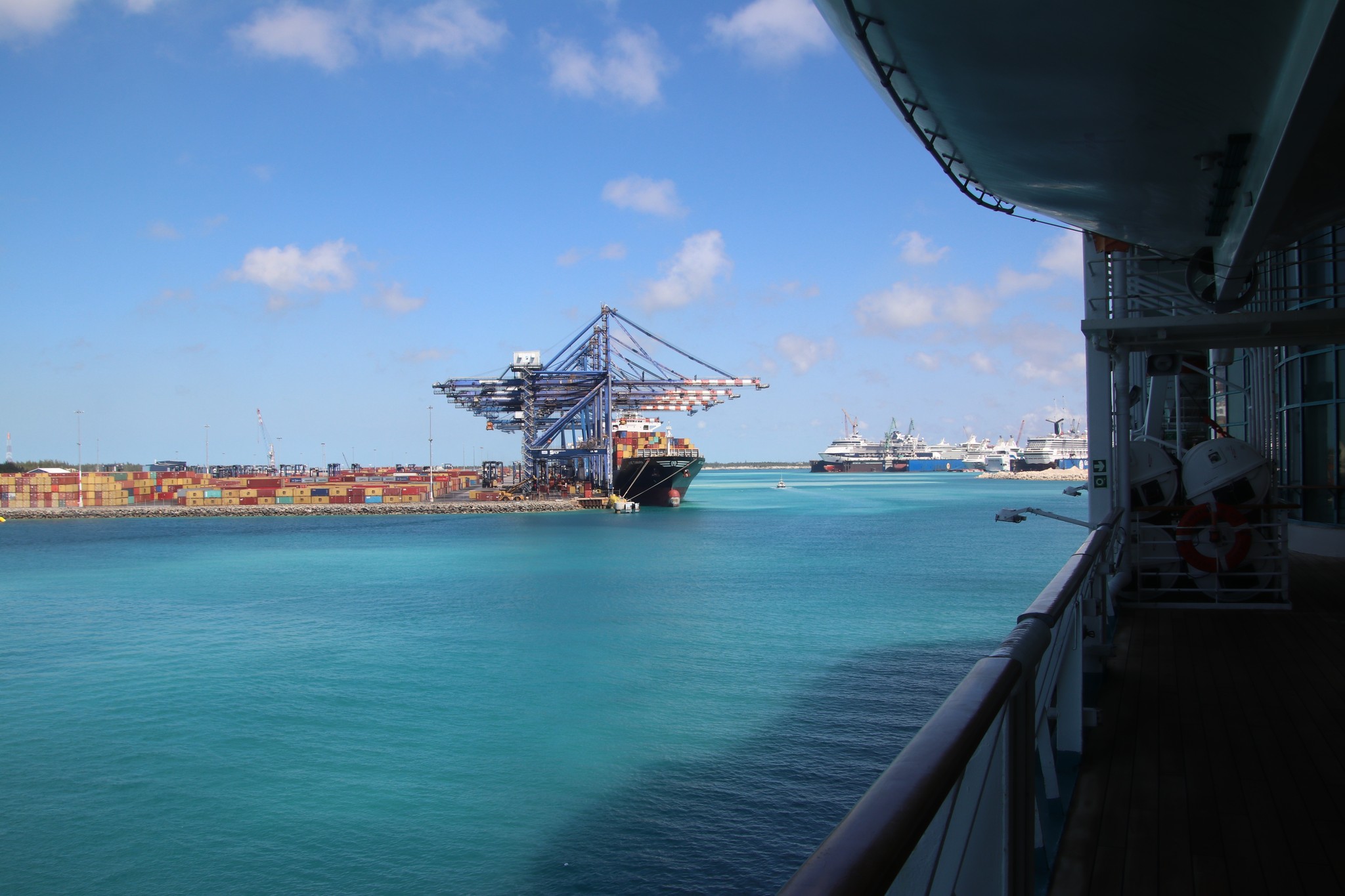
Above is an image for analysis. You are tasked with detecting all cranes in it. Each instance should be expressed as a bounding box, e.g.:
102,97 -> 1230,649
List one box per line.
342,452 -> 350,469
432,299 -> 770,489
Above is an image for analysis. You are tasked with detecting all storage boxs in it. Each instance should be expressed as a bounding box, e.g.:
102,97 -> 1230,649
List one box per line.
611,431 -> 694,465
0,466 -> 593,507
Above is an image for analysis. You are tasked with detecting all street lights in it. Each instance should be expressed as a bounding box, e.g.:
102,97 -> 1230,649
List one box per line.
473,446 -> 475,471
73,408 -> 85,507
352,447 -> 355,464
427,405 -> 434,502
276,437 -> 282,477
321,442 -> 325,477
204,424 -> 211,473
97,439 -> 101,472
462,443 -> 465,471
405,452 -> 407,469
373,449 -> 376,472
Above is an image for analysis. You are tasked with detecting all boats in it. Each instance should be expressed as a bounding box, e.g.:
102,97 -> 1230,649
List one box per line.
613,418 -> 707,507
809,430 -> 1088,473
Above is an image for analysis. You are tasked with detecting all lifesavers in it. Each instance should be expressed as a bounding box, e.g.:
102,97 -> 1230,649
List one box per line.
1175,502 -> 1252,574
